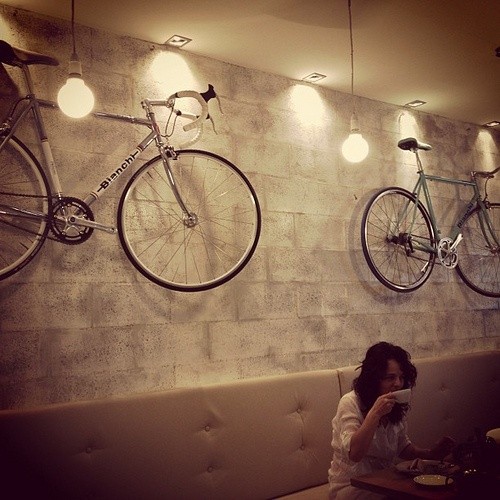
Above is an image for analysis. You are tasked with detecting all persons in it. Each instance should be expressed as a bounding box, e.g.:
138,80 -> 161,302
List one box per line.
328,342 -> 456,500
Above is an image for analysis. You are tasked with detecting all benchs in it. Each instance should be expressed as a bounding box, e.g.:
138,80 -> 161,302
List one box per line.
336,350 -> 500,464
0,368 -> 342,500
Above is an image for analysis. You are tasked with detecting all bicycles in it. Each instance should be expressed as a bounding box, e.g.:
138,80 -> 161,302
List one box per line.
360,134 -> 500,300
0,37 -> 263,292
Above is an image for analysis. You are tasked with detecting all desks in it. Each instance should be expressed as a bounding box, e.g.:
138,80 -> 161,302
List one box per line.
350,453 -> 458,500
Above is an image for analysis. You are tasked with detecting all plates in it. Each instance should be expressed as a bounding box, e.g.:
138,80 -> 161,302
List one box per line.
414,475 -> 451,487
396,460 -> 459,476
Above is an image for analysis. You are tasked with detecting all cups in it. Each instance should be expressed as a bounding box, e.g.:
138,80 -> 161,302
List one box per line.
389,390 -> 412,403
